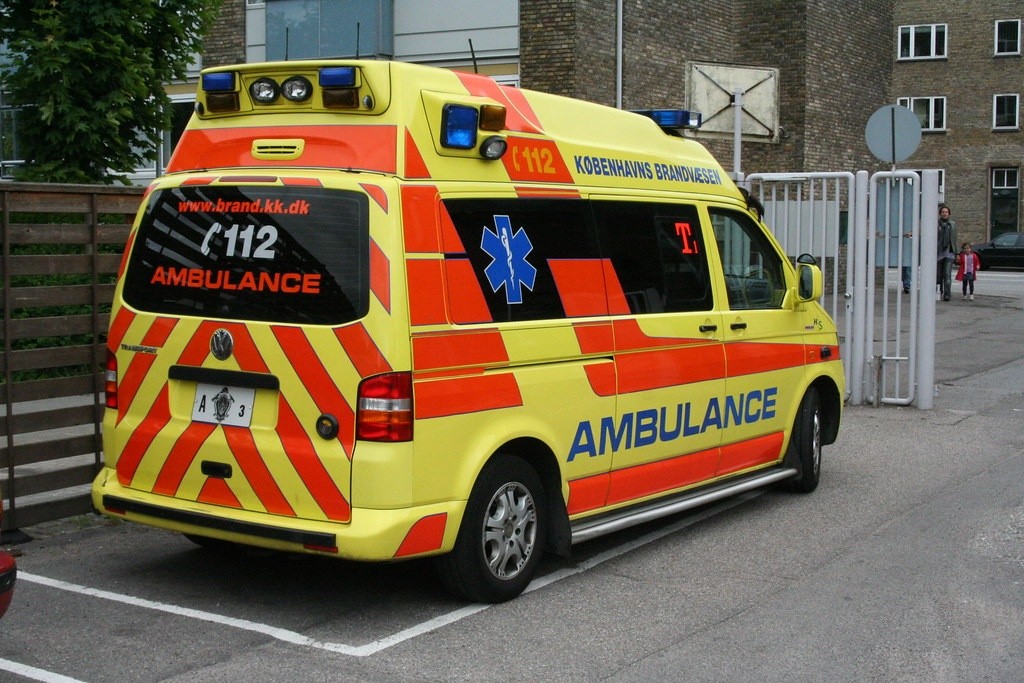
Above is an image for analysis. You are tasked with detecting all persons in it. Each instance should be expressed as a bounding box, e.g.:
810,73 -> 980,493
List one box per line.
902,230 -> 912,294
936,207 -> 960,301
955,243 -> 980,301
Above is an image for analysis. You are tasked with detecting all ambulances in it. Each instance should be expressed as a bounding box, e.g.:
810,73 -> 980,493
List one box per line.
90,59 -> 850,605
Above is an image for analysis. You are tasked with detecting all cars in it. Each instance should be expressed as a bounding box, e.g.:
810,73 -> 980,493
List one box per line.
961,231 -> 1024,271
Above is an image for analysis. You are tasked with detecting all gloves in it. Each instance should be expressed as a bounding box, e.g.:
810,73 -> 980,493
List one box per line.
966,272 -> 973,281
963,274 -> 967,281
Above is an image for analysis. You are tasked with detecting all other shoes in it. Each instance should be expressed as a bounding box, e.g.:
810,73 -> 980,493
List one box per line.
962,296 -> 967,299
944,295 -> 950,301
903,288 -> 909,294
969,295 -> 974,300
